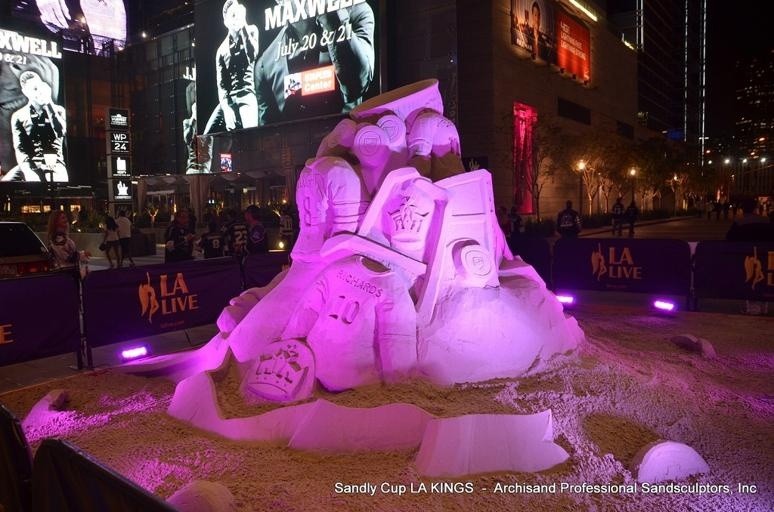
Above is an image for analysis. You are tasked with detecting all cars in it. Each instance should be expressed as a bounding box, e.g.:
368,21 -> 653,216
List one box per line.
0,222 -> 53,280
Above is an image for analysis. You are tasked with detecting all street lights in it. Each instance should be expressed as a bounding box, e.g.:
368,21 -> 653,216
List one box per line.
674,175 -> 677,215
578,159 -> 585,218
631,166 -> 635,204
723,157 -> 766,205
45,170 -> 55,217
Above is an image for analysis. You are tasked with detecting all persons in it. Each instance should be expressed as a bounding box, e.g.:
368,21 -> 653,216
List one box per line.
116,212 -> 136,267
613,197 -> 623,235
556,201 -> 582,237
499,206 -> 510,237
0,52 -> 60,172
253,0 -> 374,124
696,195 -> 772,225
205,2 -> 260,135
100,215 -> 122,269
1,0 -> 96,54
3,70 -> 67,183
182,104 -> 214,175
511,207 -> 524,234
628,203 -> 640,233
517,1 -> 552,60
160,200 -> 295,262
47,210 -> 93,270
